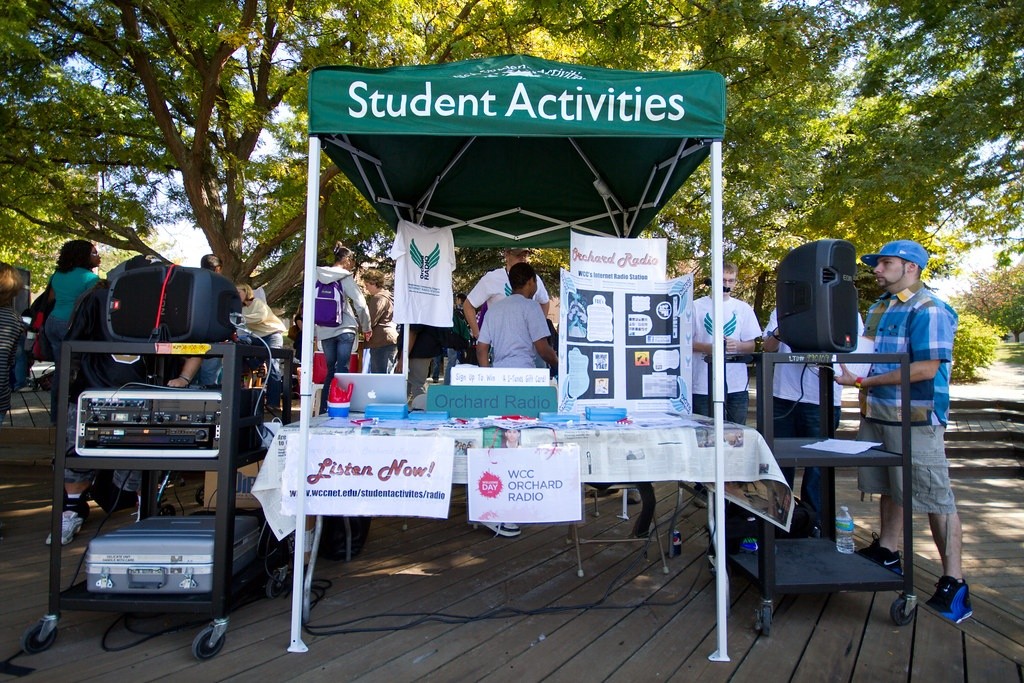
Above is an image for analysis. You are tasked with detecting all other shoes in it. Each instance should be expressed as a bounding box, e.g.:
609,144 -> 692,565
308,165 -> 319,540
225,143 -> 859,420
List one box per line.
433,379 -> 438,382
694,495 -> 707,509
809,527 -> 820,539
628,489 -> 642,504
264,404 -> 281,413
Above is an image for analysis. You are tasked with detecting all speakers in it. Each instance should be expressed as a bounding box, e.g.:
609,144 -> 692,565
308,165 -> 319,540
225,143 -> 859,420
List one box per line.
775,237 -> 858,353
102,265 -> 242,343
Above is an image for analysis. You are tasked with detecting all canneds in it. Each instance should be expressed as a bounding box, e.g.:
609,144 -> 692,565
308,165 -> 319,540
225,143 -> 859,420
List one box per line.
672,531 -> 682,556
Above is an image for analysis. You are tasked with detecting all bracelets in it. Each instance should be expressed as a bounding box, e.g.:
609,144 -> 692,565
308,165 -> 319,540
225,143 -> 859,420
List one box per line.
179,374 -> 191,384
855,377 -> 864,391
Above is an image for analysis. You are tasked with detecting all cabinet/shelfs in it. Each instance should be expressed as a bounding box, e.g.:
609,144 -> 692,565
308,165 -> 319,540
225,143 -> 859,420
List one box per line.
705,346 -> 919,632
20,342 -> 295,660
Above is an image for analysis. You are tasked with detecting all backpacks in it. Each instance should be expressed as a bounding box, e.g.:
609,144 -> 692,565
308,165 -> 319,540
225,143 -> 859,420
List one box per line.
314,279 -> 343,327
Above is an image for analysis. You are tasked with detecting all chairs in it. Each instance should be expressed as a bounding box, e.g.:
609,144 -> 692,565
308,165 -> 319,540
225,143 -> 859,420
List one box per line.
566,481 -> 669,576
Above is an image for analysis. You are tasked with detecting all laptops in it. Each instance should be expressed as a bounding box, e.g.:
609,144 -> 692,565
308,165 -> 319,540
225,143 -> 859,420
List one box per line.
335,373 -> 407,413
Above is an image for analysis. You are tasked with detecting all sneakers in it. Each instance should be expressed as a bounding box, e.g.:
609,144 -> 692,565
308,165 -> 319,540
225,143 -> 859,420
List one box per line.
46,511 -> 83,545
925,575 -> 973,624
481,523 -> 521,538
854,532 -> 904,575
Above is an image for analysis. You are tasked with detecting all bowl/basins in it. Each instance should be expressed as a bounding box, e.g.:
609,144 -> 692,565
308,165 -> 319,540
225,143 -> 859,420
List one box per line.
327,401 -> 350,416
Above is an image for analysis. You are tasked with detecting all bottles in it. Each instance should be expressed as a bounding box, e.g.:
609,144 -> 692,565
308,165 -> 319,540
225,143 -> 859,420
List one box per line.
836,506 -> 855,554
674,526 -> 681,556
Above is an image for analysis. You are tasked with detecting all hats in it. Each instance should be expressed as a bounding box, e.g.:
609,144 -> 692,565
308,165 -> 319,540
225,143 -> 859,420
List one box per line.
504,247 -> 532,256
860,240 -> 929,270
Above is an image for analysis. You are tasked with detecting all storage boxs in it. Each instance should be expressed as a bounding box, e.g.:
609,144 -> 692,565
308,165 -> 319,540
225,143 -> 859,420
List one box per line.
205,451 -> 266,511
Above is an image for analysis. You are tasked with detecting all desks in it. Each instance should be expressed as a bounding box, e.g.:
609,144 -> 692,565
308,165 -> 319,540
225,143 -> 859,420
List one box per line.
251,415 -> 794,623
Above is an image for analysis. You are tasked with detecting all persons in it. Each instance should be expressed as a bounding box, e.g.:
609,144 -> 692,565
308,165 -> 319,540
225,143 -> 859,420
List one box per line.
293,241 -> 642,537
692,262 -> 762,506
0,239 -> 302,544
763,293 -> 865,519
835,240 -> 973,625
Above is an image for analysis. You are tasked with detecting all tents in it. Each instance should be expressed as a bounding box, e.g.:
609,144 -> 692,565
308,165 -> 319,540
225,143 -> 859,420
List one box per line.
285,55 -> 731,662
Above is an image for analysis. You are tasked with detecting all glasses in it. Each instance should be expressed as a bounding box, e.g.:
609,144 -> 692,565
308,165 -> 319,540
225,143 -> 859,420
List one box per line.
90,251 -> 98,256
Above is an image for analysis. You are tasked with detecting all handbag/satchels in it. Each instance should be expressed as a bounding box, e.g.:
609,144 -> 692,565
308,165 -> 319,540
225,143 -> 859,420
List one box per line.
725,496 -> 816,538
